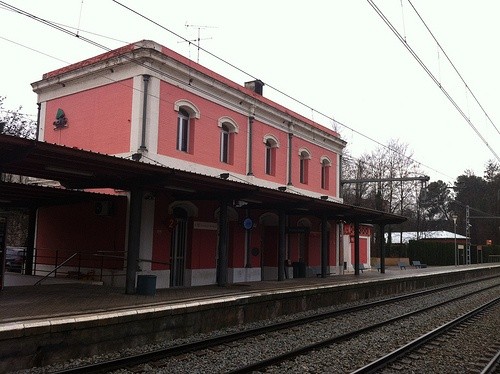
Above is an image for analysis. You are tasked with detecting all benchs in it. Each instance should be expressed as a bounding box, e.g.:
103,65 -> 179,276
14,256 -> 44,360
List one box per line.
375,263 -> 381,271
354,263 -> 368,275
412,261 -> 424,269
399,262 -> 408,270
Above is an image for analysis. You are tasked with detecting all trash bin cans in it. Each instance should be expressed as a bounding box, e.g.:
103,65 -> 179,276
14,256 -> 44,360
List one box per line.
292,261 -> 307,278
136,274 -> 158,296
